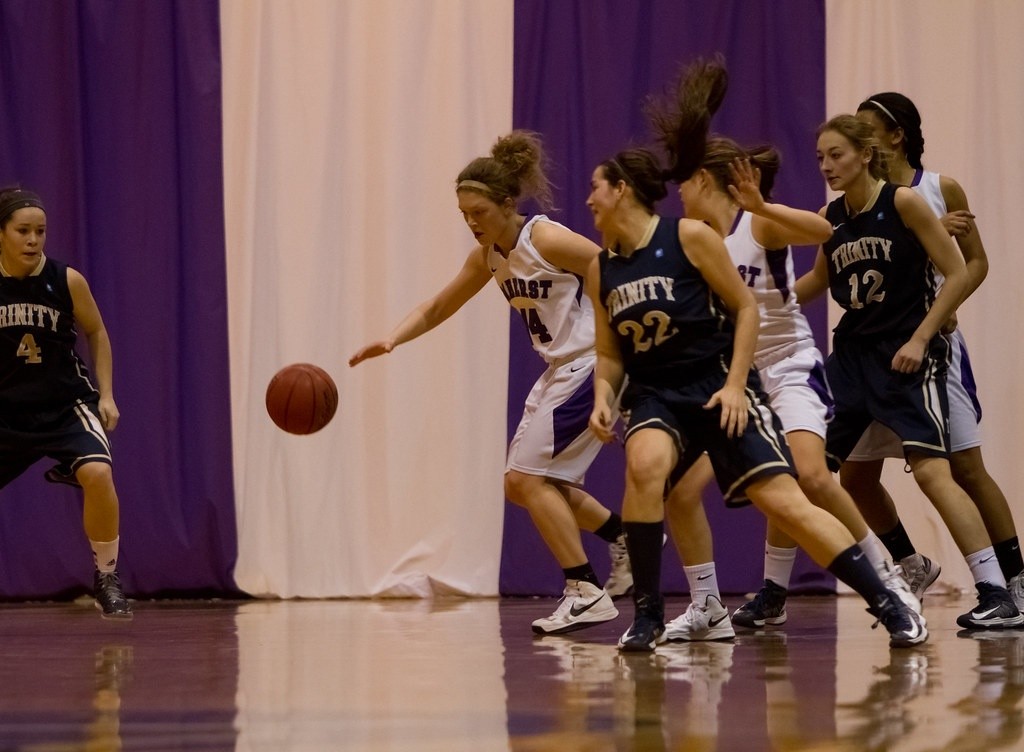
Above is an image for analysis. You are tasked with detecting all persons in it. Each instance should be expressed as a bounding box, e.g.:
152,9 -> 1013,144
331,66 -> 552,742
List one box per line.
349,132 -> 669,633
1,187 -> 134,618
586,57 -> 929,652
666,138 -> 924,641
841,92 -> 1024,615
732,117 -> 1024,629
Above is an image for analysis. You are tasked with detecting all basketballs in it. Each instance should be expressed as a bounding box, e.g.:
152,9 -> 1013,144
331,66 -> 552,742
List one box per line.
265,362 -> 339,435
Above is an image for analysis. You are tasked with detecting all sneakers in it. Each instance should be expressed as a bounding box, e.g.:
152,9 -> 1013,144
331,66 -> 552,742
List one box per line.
957,582 -> 1024,628
617,591 -> 668,650
731,579 -> 787,630
94,569 -> 134,621
532,579 -> 619,635
865,589 -> 928,648
602,529 -> 667,602
664,594 -> 737,640
1007,568 -> 1024,613
878,563 -> 923,614
893,552 -> 941,605
